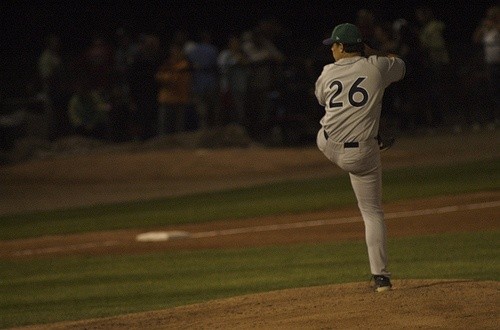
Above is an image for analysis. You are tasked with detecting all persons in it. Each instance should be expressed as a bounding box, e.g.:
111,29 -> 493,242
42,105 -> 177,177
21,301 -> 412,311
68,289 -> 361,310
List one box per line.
314,23 -> 405,291
1,5 -> 499,145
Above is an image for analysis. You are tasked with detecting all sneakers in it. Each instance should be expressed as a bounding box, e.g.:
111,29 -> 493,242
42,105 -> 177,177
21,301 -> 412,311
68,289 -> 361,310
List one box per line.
373,275 -> 393,292
378,138 -> 394,151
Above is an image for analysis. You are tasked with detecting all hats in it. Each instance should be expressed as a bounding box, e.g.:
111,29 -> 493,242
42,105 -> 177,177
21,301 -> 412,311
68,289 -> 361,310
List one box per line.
321,24 -> 363,46
